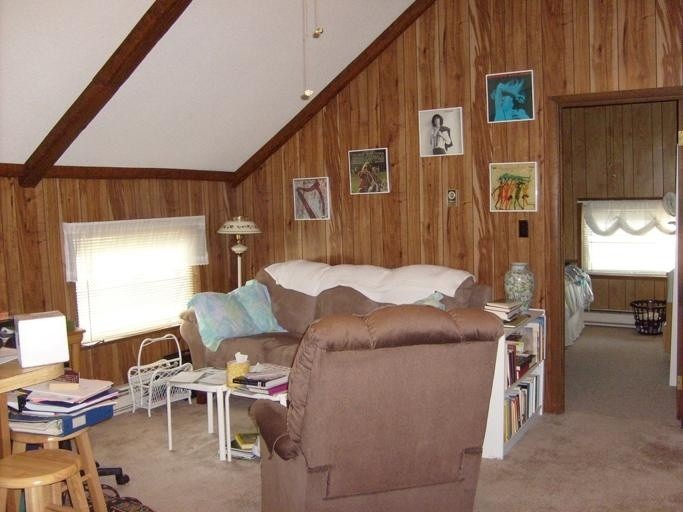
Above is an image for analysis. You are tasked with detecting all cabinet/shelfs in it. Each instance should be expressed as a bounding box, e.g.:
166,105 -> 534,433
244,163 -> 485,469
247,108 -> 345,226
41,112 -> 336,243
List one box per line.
482,309 -> 547,461
0,360 -> 64,458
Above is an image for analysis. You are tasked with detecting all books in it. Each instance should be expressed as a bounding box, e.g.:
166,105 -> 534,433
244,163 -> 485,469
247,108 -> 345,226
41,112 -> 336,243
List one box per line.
217,433 -> 260,460
482,298 -> 546,442
233,371 -> 289,396
5,368 -> 119,417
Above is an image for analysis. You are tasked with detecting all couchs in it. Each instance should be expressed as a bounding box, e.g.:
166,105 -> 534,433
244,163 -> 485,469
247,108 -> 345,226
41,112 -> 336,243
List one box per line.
180,258 -> 490,383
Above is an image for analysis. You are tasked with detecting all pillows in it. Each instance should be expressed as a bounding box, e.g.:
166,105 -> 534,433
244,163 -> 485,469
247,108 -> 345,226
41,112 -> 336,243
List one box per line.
188,278 -> 289,351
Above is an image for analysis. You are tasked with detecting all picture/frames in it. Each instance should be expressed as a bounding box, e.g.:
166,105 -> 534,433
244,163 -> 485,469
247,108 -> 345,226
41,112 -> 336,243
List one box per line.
418,107 -> 464,158
486,70 -> 535,123
489,162 -> 538,213
348,147 -> 390,195
293,177 -> 331,221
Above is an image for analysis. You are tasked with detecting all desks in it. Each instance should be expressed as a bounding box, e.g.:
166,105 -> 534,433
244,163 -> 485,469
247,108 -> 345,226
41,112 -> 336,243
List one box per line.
166,366 -> 287,462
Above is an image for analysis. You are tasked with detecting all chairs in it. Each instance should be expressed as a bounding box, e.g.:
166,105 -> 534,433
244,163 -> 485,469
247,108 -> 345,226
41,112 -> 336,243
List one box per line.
250,303 -> 504,510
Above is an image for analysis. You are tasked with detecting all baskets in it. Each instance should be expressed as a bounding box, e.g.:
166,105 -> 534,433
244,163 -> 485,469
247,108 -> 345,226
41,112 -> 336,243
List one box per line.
629,298 -> 665,336
128,334 -> 193,418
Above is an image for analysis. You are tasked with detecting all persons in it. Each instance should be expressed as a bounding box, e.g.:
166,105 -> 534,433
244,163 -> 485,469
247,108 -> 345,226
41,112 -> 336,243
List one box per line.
430,114 -> 453,155
357,162 -> 382,193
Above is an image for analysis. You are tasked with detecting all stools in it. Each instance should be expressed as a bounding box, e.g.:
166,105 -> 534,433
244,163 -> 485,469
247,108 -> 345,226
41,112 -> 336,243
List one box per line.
0,449 -> 91,512
11,431 -> 107,512
